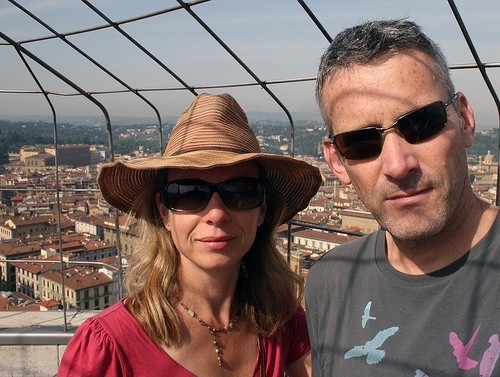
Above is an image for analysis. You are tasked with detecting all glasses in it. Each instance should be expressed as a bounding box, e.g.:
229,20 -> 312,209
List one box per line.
157,175 -> 270,214
322,93 -> 459,162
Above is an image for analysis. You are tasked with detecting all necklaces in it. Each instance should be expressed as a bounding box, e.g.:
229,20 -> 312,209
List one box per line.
168,292 -> 246,367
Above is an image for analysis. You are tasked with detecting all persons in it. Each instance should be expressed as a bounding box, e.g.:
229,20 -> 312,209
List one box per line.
302,19 -> 499,377
56,93 -> 323,376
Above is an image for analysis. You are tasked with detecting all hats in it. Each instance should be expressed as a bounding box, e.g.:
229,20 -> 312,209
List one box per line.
97,92 -> 323,232
